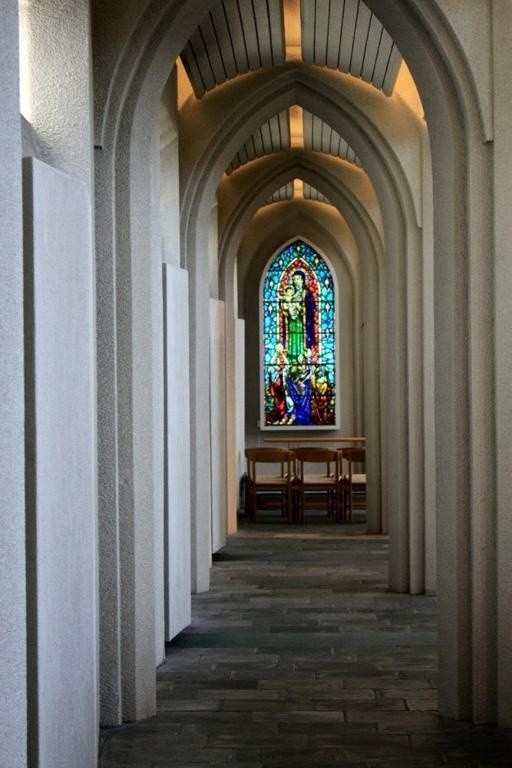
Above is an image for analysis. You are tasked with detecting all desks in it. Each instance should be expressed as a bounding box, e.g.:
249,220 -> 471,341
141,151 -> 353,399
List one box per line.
262,436 -> 367,477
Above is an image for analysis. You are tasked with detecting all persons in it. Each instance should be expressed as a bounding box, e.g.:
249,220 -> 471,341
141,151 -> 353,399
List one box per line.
282,270 -> 316,362
277,285 -> 304,316
265,342 -> 332,425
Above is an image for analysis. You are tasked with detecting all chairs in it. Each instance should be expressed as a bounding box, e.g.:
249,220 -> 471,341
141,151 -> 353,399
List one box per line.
243,445 -> 366,524
